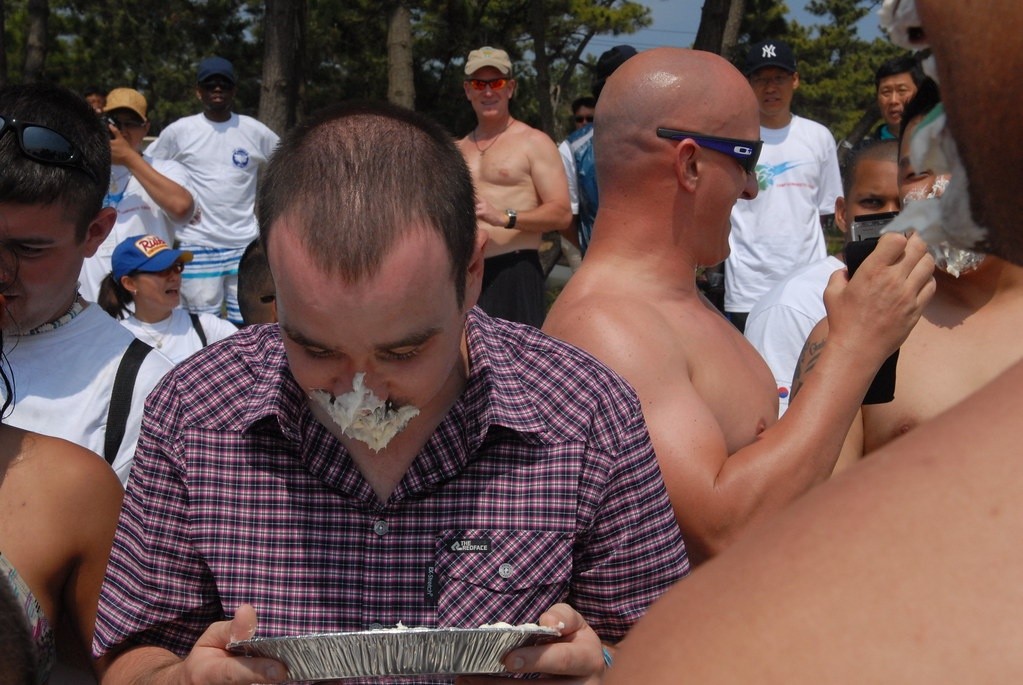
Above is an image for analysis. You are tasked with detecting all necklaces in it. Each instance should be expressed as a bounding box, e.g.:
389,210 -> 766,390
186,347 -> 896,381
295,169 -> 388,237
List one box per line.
474,117 -> 515,155
7,279 -> 85,338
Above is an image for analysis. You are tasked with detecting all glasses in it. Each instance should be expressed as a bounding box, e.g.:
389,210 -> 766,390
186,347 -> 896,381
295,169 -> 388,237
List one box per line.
0,117 -> 102,186
655,126 -> 764,174
203,78 -> 233,90
576,115 -> 594,122
108,117 -> 141,128
470,76 -> 510,90
137,260 -> 184,273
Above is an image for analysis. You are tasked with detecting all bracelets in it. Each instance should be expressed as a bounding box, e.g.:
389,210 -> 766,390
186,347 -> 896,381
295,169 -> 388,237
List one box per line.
601,645 -> 612,672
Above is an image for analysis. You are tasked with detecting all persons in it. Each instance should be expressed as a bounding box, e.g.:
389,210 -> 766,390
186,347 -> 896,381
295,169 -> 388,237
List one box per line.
98,97 -> 693,685
536,49 -> 937,568
0,1 -> 1023,685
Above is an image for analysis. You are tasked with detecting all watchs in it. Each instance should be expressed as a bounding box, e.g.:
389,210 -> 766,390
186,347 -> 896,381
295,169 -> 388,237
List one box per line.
506,208 -> 517,229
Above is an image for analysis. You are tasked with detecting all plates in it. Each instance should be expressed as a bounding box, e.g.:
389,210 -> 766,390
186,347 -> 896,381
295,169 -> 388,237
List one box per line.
225,627 -> 563,682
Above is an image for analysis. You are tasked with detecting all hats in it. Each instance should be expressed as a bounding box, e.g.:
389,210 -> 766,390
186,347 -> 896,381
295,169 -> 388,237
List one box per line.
198,56 -> 238,84
598,44 -> 637,79
112,234 -> 194,284
464,46 -> 513,77
104,86 -> 147,122
744,40 -> 796,74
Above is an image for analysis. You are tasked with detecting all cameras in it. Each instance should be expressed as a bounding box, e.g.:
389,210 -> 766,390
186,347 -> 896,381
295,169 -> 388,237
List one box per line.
102,115 -> 122,139
